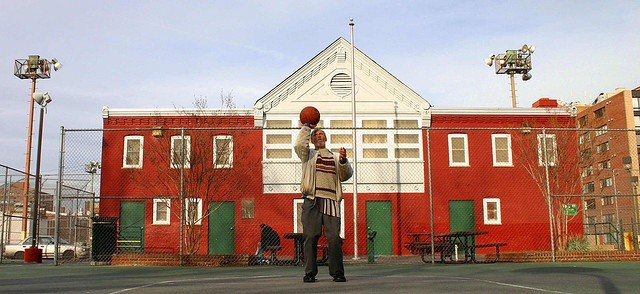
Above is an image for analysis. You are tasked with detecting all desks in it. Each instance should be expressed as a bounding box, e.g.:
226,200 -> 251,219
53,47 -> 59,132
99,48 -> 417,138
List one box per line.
403,232 -> 431,242
283,232 -> 321,265
434,230 -> 490,262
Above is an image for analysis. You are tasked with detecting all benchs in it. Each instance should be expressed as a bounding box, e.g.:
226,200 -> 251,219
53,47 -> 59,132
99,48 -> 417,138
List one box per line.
467,241 -> 507,261
316,244 -> 328,266
414,244 -> 454,261
402,241 -> 433,255
267,245 -> 283,264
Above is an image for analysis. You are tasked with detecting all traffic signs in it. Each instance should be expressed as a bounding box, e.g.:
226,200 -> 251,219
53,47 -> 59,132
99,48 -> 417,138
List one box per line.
562,204 -> 576,215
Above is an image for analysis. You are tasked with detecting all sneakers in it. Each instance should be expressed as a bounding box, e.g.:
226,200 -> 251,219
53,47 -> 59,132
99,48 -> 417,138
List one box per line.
333,276 -> 346,282
304,275 -> 315,282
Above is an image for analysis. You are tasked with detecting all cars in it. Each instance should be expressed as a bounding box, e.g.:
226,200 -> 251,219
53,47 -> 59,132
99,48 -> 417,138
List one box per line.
3,235 -> 88,262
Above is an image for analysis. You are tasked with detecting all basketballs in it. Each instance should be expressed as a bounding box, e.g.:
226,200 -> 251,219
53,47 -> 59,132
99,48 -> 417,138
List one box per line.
300,106 -> 320,124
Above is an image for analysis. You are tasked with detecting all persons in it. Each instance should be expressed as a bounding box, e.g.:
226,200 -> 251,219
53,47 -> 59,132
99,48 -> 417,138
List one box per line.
294,122 -> 354,282
255,223 -> 280,263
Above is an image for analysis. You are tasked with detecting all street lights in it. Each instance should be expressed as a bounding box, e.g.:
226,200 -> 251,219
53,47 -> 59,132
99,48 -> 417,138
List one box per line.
20,55 -> 62,243
85,161 -> 101,258
31,91 -> 51,248
629,175 -> 640,235
485,43 -> 535,109
622,156 -> 638,235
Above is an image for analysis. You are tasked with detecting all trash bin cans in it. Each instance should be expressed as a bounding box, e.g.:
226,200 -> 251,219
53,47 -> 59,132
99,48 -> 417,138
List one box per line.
90,216 -> 119,261
